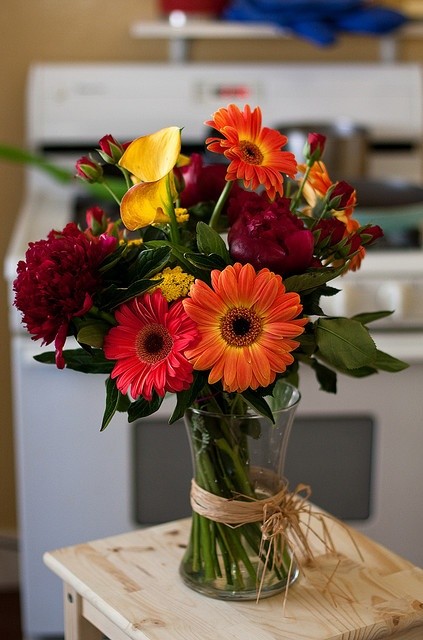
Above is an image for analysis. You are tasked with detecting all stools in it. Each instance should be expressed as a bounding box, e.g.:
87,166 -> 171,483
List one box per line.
42,498 -> 423,640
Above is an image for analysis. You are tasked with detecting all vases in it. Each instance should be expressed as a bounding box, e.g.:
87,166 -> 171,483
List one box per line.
178,382 -> 301,600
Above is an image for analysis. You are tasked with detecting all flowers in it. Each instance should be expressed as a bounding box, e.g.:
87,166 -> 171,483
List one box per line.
13,103 -> 410,593
